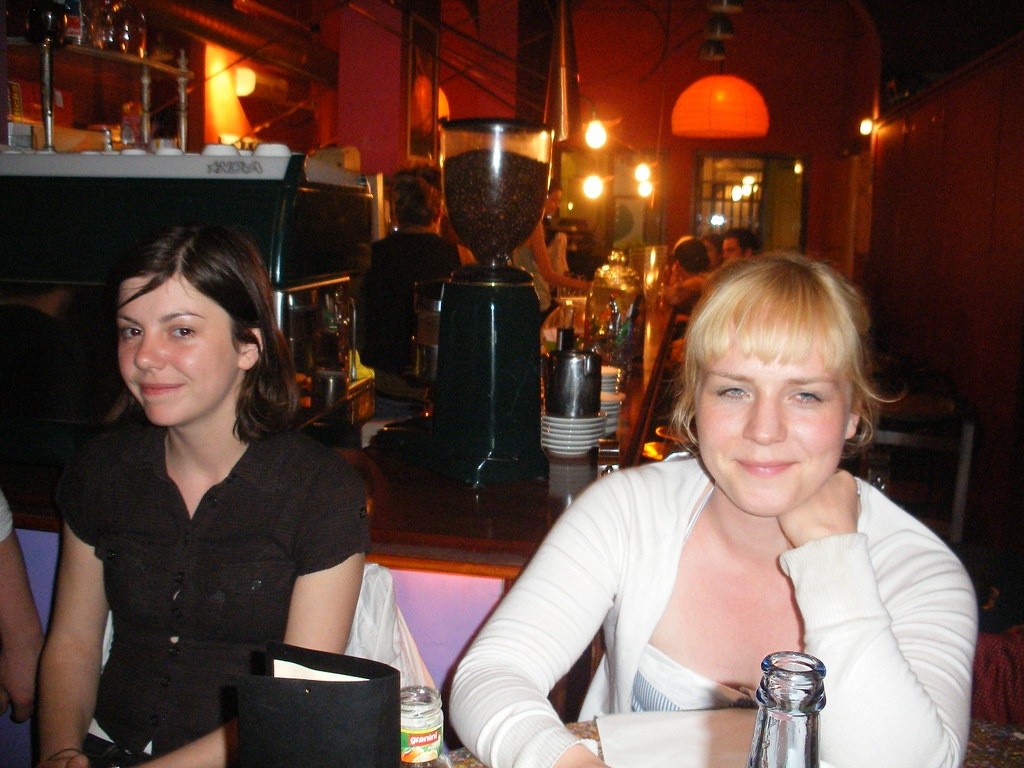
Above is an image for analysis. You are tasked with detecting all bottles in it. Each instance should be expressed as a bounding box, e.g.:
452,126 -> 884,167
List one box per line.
746,652 -> 826,768
132,12 -> 147,54
95,0 -> 113,50
116,18 -> 130,52
400,685 -> 454,768
584,250 -> 646,378
62,0 -> 83,46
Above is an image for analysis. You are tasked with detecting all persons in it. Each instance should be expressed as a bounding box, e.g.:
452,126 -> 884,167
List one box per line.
654,227 -> 766,424
358,174 -> 464,375
34,212 -> 372,768
448,246 -> 977,768
510,185 -> 593,316
0,490 -> 45,725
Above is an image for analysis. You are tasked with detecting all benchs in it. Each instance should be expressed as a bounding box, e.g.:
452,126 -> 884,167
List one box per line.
0,514 -> 509,768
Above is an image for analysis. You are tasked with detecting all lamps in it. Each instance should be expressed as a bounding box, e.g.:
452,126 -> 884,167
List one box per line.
697,41 -> 730,59
705,14 -> 734,42
670,59 -> 769,140
704,0 -> 744,16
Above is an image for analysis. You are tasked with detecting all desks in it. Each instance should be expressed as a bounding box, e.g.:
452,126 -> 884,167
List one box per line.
406,713 -> 1024,768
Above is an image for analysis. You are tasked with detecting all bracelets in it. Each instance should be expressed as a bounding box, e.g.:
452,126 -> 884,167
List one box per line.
47,747 -> 82,761
571,226 -> 577,232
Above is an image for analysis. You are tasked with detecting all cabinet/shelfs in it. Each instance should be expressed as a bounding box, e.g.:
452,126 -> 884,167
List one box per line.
1,152 -> 374,435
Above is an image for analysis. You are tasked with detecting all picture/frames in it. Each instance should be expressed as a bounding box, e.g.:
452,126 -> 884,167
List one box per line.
406,11 -> 440,165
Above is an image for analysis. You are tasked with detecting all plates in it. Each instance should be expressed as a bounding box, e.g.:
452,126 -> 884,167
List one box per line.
595,392 -> 622,437
541,416 -> 605,456
599,366 -> 621,391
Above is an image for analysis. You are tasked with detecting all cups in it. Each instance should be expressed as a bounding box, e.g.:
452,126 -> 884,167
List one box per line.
79,144 -> 290,157
541,348 -> 602,417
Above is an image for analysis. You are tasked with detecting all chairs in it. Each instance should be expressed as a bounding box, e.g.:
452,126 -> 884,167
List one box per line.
852,387 -> 977,548
